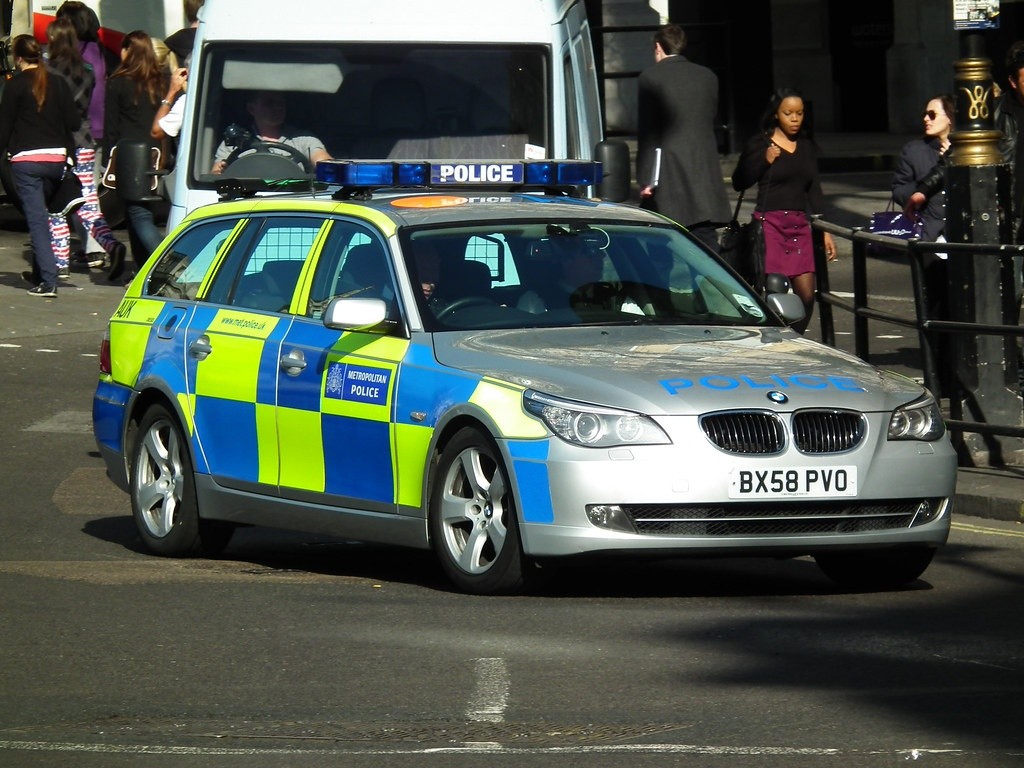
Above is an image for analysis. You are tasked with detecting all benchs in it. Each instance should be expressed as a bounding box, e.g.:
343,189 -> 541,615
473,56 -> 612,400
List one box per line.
234,245 -> 297,307
291,81 -> 529,158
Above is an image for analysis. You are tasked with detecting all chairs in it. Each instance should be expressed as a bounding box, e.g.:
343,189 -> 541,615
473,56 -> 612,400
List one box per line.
335,243 -> 384,315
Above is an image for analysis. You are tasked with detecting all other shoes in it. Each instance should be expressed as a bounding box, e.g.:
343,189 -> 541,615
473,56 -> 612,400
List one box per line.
21,270 -> 40,286
72,252 -> 105,269
26,279 -> 58,297
109,244 -> 126,281
57,268 -> 70,278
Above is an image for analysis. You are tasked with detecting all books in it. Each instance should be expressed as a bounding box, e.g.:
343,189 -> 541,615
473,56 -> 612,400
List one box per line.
650,148 -> 661,189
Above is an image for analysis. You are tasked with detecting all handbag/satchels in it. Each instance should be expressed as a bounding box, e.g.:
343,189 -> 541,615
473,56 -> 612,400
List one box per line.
720,216 -> 765,294
865,194 -> 922,265
48,170 -> 87,216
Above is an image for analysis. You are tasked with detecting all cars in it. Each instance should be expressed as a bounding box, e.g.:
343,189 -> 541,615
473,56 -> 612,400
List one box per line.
91,158 -> 959,596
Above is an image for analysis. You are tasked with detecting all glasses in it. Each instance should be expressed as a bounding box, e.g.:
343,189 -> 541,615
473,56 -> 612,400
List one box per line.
920,110 -> 949,120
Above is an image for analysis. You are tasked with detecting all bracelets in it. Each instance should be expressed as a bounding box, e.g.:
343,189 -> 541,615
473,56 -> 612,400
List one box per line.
161,100 -> 172,107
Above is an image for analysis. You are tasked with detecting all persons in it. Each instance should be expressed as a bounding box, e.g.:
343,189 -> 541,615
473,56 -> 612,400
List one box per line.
891,40 -> 1024,397
210,90 -> 333,174
731,88 -> 836,337
1,0 -> 202,297
410,243 -> 507,320
638,24 -> 732,254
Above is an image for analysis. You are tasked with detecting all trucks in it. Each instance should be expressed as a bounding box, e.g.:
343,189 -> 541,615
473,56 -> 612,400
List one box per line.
112,0 -> 634,284
